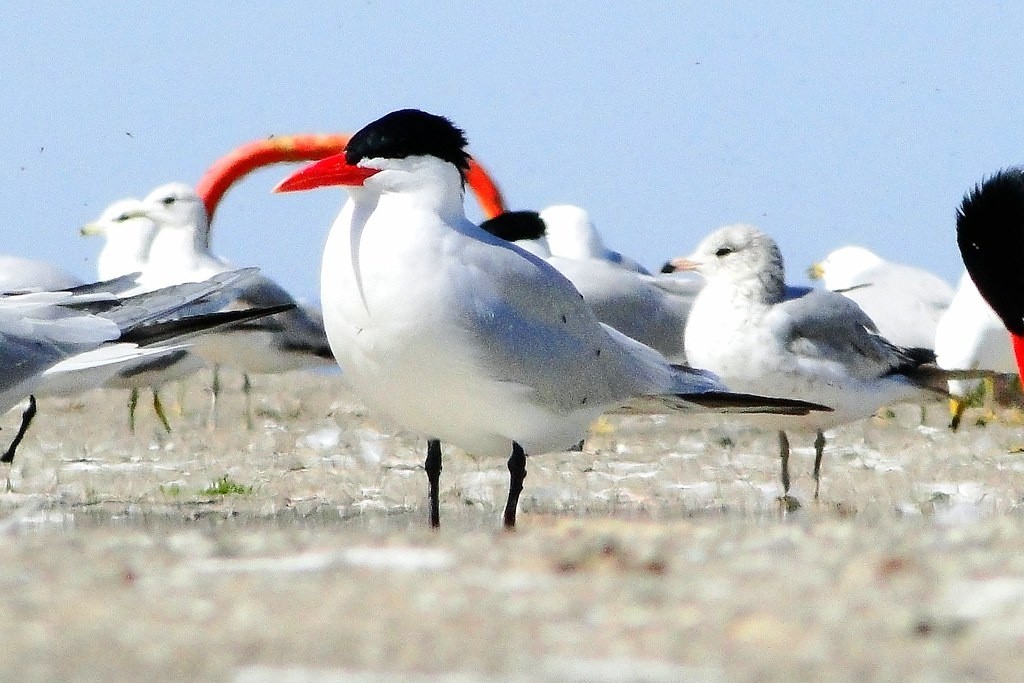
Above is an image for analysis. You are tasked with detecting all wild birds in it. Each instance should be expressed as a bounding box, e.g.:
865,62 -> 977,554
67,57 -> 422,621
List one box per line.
1,179 -> 341,493
655,224 -> 1024,501
271,109 -> 833,529
